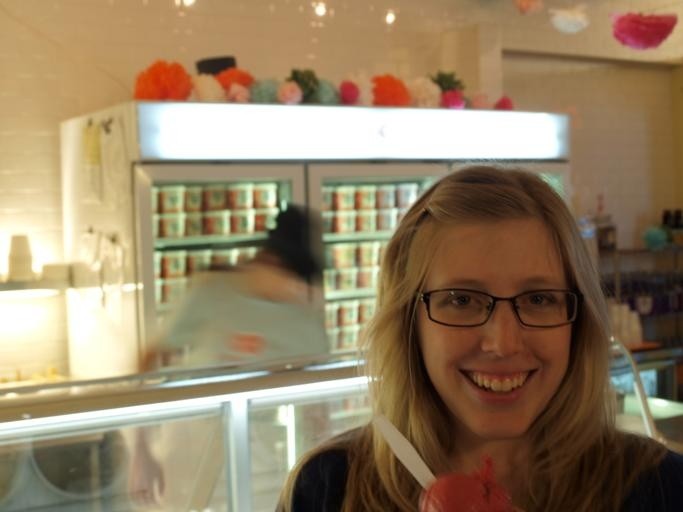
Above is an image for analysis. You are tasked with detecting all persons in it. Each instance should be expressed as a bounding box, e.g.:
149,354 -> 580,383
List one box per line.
275,166 -> 683,512
127,202 -> 332,512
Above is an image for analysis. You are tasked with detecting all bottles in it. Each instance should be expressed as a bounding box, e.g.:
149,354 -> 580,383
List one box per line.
7,233 -> 34,284
591,213 -> 617,251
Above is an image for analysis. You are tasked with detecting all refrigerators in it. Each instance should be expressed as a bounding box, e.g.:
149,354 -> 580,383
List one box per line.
55,98 -> 575,391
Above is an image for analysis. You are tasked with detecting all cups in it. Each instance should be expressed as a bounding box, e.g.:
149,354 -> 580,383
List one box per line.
636,295 -> 653,315
39,262 -> 95,282
609,301 -> 644,345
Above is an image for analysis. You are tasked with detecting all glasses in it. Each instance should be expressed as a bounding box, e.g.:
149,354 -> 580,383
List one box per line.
416,289 -> 583,327
